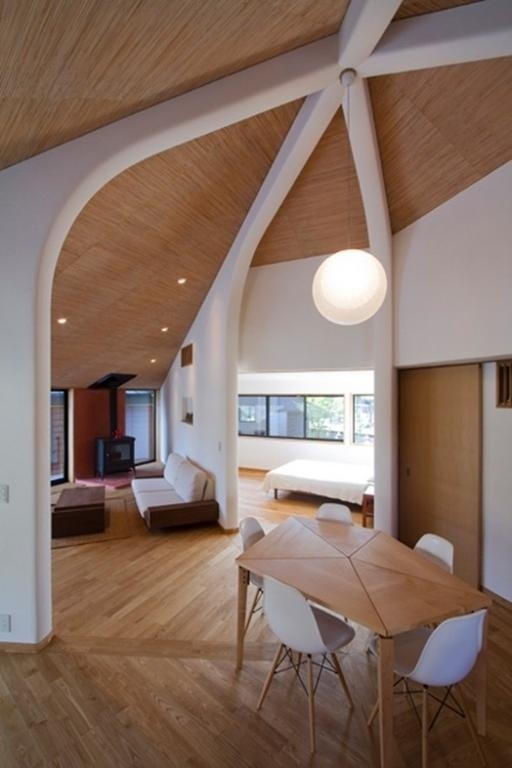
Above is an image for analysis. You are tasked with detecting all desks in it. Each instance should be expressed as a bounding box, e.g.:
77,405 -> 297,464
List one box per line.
231,515 -> 492,767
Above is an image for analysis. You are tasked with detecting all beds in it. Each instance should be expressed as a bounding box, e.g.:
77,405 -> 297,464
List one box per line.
264,460 -> 375,505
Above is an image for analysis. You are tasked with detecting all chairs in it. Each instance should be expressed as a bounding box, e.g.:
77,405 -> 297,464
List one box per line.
408,530 -> 456,630
255,574 -> 356,754
312,501 -> 356,525
237,516 -> 265,636
368,598 -> 493,767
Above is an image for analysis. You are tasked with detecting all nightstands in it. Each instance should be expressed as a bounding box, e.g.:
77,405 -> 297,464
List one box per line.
362,486 -> 374,527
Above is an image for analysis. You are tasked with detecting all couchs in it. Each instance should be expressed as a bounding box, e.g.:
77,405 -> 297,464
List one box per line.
132,453 -> 218,531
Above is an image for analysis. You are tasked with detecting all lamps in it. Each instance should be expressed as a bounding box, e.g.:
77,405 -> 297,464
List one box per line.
312,67 -> 387,325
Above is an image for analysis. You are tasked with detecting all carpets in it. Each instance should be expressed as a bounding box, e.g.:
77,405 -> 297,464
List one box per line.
50,491 -> 133,551
77,463 -> 163,488
